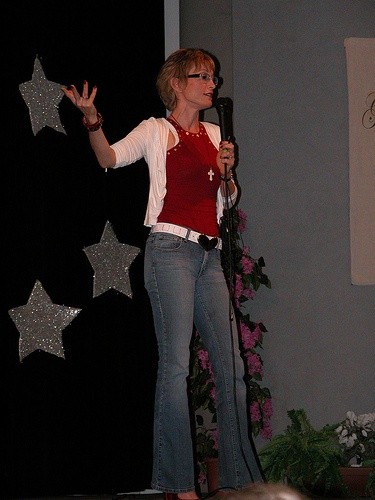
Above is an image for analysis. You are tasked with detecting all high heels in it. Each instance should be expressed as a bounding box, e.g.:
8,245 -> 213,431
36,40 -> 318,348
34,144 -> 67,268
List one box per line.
165,492 -> 201,500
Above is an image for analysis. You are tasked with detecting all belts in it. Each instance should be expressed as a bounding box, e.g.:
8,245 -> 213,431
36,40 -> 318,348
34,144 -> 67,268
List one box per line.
148,222 -> 223,251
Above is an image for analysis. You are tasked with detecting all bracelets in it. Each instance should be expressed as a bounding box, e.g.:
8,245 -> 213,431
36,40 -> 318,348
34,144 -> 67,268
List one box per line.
83,111 -> 103,131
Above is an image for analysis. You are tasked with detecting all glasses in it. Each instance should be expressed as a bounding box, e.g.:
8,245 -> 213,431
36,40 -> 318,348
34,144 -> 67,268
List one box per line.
178,71 -> 219,86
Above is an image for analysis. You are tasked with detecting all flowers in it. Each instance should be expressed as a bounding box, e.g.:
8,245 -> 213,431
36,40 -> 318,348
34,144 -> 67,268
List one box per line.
335,411 -> 375,465
181,216 -> 272,453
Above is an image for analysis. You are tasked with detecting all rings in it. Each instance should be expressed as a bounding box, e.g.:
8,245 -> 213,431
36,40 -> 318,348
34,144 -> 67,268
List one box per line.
83,96 -> 88,100
230,155 -> 232,159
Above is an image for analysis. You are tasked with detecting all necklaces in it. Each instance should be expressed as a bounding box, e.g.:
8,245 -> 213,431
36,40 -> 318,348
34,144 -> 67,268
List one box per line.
171,114 -> 180,125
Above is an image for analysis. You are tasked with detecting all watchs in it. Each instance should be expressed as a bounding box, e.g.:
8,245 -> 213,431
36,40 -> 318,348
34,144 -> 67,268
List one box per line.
219,170 -> 233,182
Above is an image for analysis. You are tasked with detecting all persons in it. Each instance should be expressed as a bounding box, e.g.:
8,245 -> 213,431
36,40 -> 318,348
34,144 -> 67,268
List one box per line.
62,49 -> 263,499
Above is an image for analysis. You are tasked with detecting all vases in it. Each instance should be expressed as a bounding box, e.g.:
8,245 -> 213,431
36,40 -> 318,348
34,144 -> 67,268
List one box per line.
332,467 -> 373,500
205,456 -> 218,496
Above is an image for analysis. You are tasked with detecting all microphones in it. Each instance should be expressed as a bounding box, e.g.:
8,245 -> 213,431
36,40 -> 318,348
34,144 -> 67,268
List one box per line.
215,96 -> 228,150
